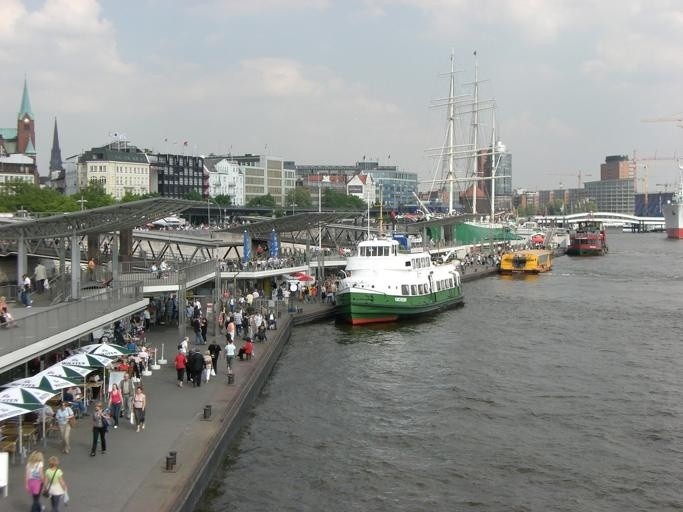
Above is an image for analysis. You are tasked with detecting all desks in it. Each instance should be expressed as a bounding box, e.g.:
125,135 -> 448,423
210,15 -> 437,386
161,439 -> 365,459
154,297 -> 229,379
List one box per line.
0,347 -> 153,464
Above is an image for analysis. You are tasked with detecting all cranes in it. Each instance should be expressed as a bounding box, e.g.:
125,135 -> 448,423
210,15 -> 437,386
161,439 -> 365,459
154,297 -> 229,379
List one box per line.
637,164 -> 649,205
542,170 -> 592,189
629,148 -> 682,194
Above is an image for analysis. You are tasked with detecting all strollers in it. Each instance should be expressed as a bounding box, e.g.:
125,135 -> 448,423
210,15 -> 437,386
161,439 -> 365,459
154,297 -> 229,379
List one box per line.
4,313 -> 15,328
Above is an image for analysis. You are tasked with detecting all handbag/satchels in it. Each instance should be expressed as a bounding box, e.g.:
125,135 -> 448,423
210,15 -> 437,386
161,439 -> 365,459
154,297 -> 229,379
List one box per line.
42,489 -> 49,497
130,411 -> 137,425
101,415 -> 115,426
32,470 -> 41,477
69,417 -> 77,426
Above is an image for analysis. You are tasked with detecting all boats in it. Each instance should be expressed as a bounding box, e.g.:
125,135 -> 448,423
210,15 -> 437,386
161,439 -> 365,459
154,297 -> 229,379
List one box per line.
660,148 -> 683,239
565,223 -> 609,256
332,180 -> 467,325
499,249 -> 553,274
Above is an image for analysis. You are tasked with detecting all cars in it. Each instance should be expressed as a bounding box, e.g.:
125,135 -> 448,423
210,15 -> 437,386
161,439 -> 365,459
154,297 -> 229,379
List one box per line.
387,208 -> 450,225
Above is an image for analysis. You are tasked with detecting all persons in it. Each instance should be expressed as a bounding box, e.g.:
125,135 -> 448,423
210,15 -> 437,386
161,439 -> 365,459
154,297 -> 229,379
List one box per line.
144,287 -> 222,388
45,456 -> 67,510
151,259 -> 178,279
26,450 -> 45,511
56,402 -> 77,454
89,401 -> 110,456
107,373 -> 147,433
19,273 -> 34,307
103,242 -> 108,255
98,312 -> 153,385
33,262 -> 47,294
1,296 -> 16,328
206,242 -> 350,373
88,257 -> 96,281
465,243 -> 546,268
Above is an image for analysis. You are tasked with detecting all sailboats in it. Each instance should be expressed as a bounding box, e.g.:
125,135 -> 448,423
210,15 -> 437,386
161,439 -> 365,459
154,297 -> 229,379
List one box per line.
395,48 -> 570,250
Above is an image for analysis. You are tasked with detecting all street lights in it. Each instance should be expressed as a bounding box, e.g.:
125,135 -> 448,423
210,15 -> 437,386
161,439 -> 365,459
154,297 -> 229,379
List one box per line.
76,195 -> 87,211
314,181 -> 324,249
206,198 -> 213,228
222,207 -> 229,230
